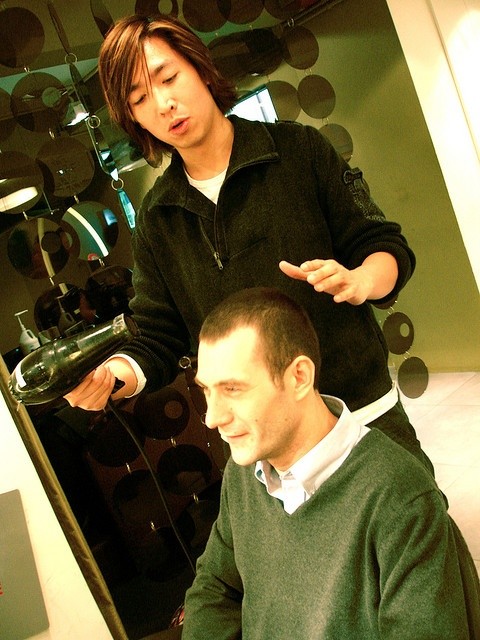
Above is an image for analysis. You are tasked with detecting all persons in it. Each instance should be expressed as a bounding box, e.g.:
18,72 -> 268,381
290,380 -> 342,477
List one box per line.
182,288 -> 480,640
63,14 -> 436,482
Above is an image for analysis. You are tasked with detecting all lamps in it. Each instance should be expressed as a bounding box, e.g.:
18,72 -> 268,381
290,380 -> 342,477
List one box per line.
61,95 -> 90,129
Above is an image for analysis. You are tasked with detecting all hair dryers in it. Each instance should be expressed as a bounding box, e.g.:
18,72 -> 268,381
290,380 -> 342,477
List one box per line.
9,314 -> 141,408
